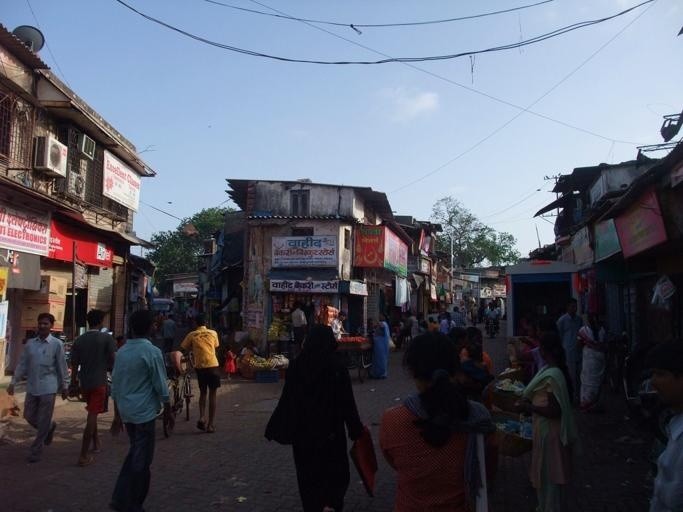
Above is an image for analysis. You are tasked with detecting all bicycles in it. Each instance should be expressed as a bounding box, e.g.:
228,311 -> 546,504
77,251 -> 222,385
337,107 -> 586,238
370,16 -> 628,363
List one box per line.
162,352 -> 193,438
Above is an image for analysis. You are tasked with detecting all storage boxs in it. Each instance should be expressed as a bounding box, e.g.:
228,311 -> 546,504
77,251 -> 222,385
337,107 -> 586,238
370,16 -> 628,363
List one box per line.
22,275 -> 67,304
19,302 -> 64,332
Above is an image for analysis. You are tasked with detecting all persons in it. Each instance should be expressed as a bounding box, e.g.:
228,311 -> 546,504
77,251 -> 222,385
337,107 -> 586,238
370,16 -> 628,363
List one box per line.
439,312 -> 456,335
485,302 -> 498,329
8,312 -> 67,462
459,328 -> 491,373
238,341 -> 255,365
516,335 -> 577,511
557,296 -> 585,383
329,312 -> 347,341
471,304 -> 478,324
368,316 -> 389,379
429,316 -> 437,332
519,302 -> 552,356
181,317 -> 220,432
290,303 -> 309,349
159,315 -> 178,352
650,342 -> 683,511
579,311 -> 609,406
452,307 -> 466,328
225,345 -> 236,378
380,332 -> 496,512
67,309 -> 116,466
264,326 -> 363,511
395,311 -> 413,348
110,310 -> 174,511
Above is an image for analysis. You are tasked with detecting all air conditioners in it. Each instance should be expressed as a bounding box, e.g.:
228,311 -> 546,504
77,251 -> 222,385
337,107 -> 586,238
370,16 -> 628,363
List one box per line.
57,169 -> 85,203
33,135 -> 66,179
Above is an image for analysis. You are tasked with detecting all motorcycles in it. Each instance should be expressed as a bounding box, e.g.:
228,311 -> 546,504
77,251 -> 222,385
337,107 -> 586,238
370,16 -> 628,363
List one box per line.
485,318 -> 499,338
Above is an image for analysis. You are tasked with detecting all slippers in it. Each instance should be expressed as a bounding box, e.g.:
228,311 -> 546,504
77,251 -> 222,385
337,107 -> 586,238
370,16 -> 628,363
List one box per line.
93,439 -> 104,453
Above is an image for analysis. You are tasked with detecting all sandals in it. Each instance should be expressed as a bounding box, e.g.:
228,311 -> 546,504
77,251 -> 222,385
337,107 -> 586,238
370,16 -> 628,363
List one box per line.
77,453 -> 97,466
207,425 -> 216,432
197,418 -> 205,431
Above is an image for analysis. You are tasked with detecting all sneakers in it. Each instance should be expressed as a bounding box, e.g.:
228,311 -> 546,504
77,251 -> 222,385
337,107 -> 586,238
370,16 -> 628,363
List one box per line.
45,422 -> 56,445
29,440 -> 42,462
